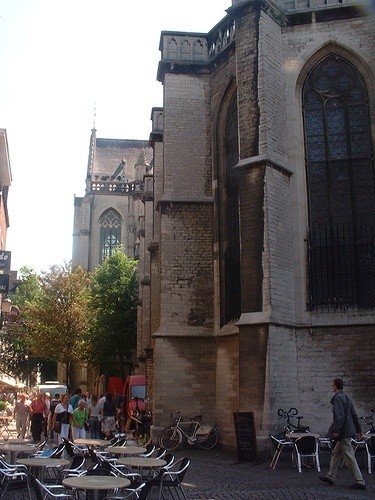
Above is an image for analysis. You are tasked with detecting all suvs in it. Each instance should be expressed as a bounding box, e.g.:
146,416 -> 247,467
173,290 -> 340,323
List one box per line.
33,381 -> 68,413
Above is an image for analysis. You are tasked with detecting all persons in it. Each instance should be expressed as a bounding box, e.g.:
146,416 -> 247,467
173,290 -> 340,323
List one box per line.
13,388 -> 124,445
319,378 -> 365,490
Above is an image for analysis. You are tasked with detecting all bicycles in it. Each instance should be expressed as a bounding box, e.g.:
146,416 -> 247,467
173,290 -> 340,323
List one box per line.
326,413 -> 375,452
276,407 -> 310,434
157,410 -> 219,451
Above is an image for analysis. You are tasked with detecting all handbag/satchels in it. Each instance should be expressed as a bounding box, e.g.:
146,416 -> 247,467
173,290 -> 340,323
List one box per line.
53,422 -> 62,433
84,418 -> 90,431
326,431 -> 337,445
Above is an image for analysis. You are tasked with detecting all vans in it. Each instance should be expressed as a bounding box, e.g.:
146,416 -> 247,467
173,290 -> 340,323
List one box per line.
127,375 -> 146,416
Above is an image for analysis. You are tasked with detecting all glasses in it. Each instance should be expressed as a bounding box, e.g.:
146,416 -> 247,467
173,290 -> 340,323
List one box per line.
332,385 -> 335,387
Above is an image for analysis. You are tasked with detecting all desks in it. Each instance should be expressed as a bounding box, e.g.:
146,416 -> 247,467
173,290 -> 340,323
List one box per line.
0,439 -> 31,445
15,458 -> 71,498
73,439 -> 111,450
0,444 -> 34,465
106,446 -> 147,458
116,457 -> 167,477
61,475 -> 131,500
285,433 -> 320,469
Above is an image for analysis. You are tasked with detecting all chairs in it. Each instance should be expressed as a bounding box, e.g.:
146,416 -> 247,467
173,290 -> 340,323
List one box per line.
0,434 -> 191,500
266,431 -> 375,474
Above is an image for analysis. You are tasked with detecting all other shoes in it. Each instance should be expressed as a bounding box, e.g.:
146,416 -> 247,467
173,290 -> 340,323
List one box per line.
320,476 -> 334,485
32,440 -> 35,444
17,434 -> 20,438
348,483 -> 365,490
36,441 -> 40,443
